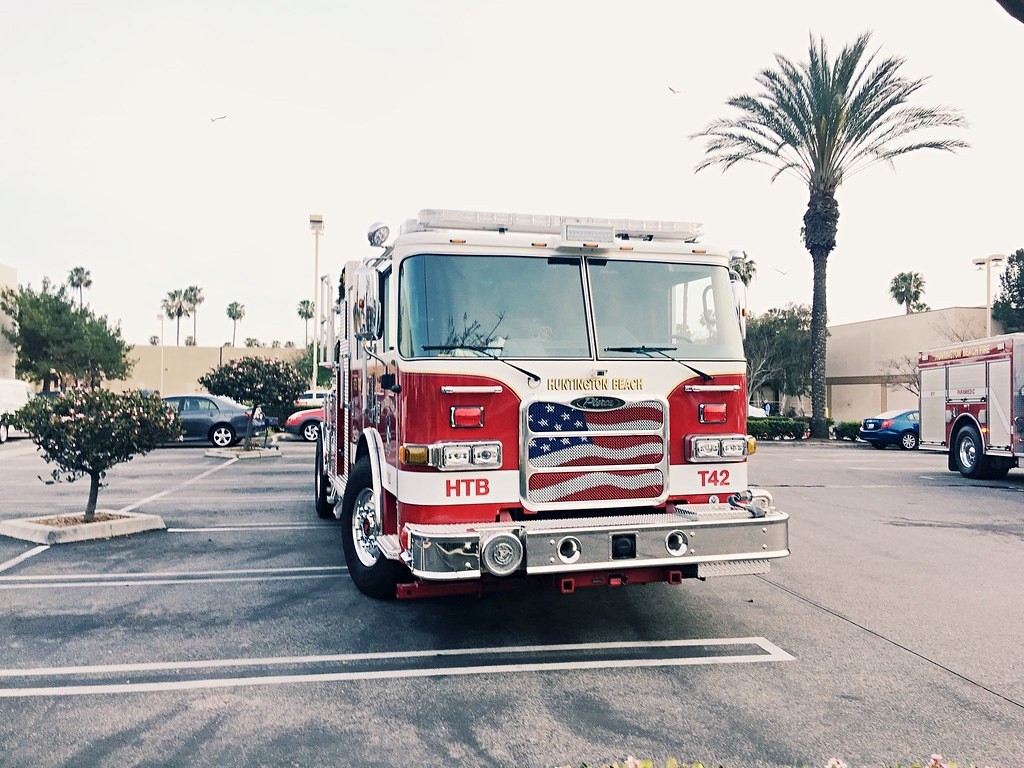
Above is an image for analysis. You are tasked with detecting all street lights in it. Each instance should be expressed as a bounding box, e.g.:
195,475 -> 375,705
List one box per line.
309,212 -> 324,407
972,253 -> 1005,338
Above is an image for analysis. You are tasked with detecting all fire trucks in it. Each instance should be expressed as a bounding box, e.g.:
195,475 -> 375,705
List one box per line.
313,211 -> 792,598
917,332 -> 1024,479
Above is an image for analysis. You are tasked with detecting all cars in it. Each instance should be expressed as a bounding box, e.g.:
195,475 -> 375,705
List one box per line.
858,408 -> 920,451
284,405 -> 330,442
139,395 -> 268,448
0,379 -> 37,444
295,391 -> 328,409
